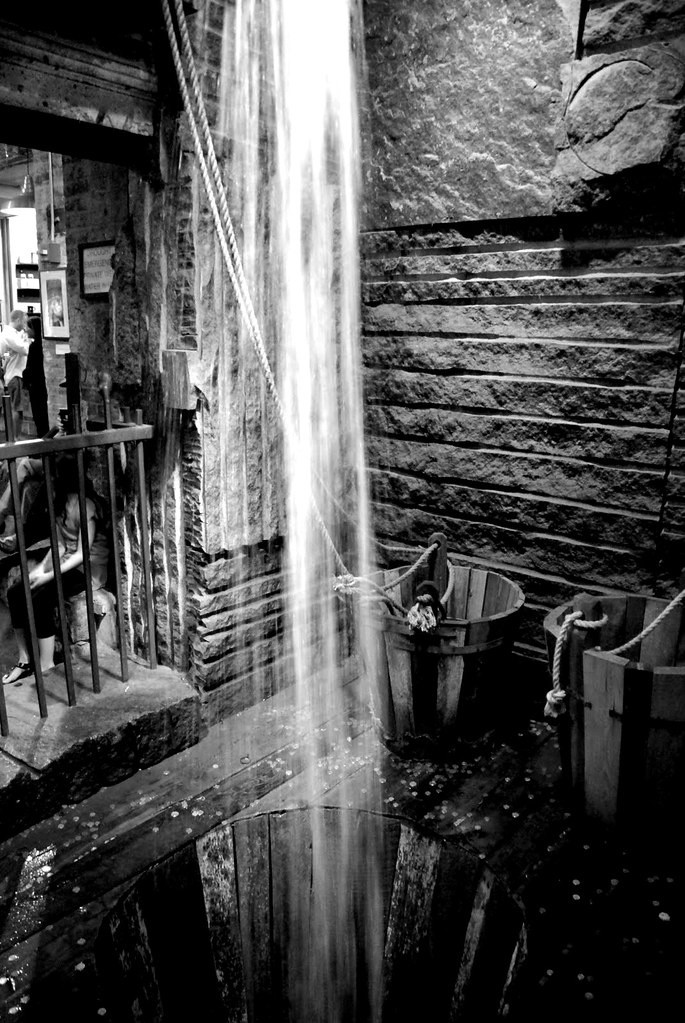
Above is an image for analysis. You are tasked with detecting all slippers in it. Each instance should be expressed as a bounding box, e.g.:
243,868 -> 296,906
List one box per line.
2,661 -> 34,684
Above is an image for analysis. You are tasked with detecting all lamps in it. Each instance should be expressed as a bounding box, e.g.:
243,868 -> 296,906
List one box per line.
22,150 -> 33,194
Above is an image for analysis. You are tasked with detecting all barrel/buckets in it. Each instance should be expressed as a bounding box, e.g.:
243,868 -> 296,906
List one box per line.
350,534 -> 526,754
543,592 -> 684,818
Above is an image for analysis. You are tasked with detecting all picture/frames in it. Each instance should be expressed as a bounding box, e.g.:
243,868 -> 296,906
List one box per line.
39,267 -> 71,340
77,233 -> 115,298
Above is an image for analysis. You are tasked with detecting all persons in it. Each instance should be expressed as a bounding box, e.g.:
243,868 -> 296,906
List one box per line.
0,449 -> 112,684
0,309 -> 50,441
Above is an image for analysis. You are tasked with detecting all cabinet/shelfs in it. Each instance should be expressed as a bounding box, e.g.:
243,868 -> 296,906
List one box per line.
14,263 -> 41,314
